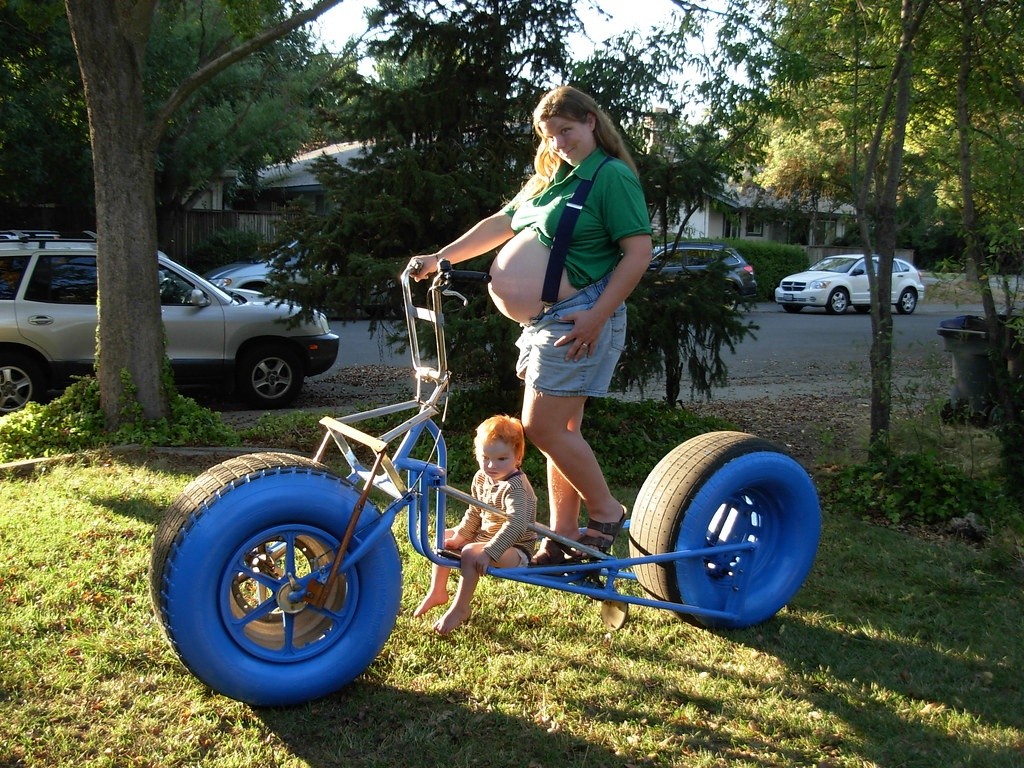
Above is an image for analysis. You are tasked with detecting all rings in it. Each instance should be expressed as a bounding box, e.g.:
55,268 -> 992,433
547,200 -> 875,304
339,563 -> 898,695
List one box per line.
583,342 -> 588,347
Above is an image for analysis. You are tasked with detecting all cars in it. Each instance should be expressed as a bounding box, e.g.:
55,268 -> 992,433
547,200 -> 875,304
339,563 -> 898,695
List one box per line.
201,235 -> 413,320
773,253 -> 924,315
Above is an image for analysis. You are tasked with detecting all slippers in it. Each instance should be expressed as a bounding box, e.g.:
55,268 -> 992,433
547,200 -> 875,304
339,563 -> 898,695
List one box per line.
574,505 -> 628,560
528,538 -> 576,566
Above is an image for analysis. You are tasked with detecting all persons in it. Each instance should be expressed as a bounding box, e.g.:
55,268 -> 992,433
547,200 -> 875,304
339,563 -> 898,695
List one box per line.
411,413 -> 538,635
400,85 -> 656,570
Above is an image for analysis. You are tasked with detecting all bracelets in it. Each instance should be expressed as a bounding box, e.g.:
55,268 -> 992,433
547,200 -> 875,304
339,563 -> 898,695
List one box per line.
432,252 -> 438,262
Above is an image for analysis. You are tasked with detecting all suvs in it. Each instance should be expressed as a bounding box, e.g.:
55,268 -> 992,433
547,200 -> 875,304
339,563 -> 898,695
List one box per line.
637,239 -> 759,314
0,226 -> 340,421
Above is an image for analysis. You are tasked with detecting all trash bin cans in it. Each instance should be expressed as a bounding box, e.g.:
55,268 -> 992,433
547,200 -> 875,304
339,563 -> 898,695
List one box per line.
934,312 -> 1024,424
